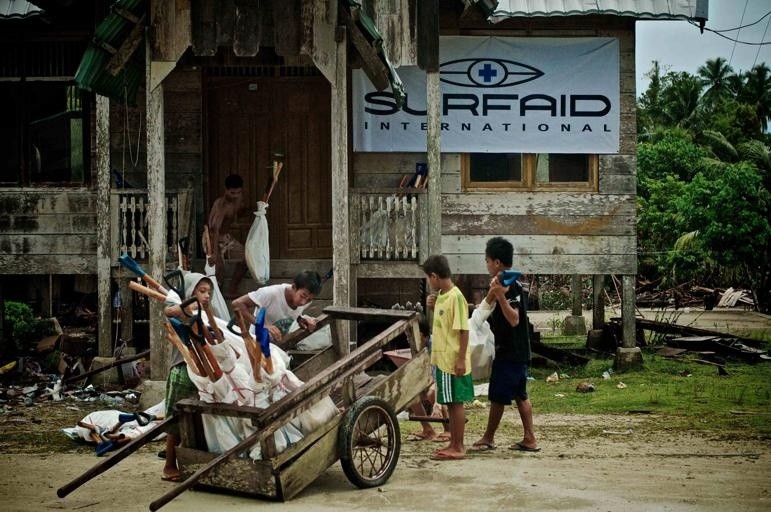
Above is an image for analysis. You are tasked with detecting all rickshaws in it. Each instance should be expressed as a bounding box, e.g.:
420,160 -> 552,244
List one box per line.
57,303 -> 437,511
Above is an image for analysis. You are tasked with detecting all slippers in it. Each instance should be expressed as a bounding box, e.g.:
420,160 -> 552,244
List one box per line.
161,471 -> 186,482
407,433 -> 541,460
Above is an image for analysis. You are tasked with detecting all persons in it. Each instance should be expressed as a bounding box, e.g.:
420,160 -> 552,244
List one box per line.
421,255 -> 475,460
403,314 -> 452,443
159,272 -> 221,482
231,269 -> 323,343
469,235 -> 542,452
200,171 -> 268,299
